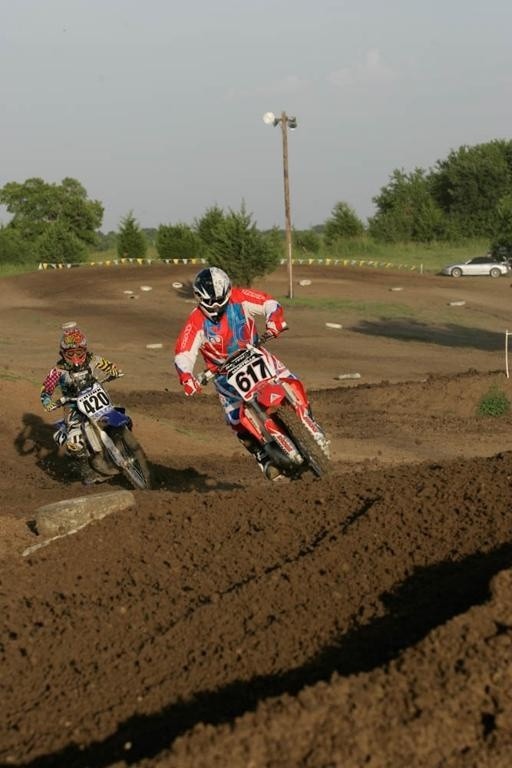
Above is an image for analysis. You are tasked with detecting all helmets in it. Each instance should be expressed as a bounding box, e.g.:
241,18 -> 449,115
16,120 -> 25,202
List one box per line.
192,266 -> 232,324
58,327 -> 88,371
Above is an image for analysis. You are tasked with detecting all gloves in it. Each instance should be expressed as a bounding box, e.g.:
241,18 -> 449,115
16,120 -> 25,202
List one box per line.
180,373 -> 202,397
266,319 -> 287,337
107,367 -> 122,378
42,398 -> 57,412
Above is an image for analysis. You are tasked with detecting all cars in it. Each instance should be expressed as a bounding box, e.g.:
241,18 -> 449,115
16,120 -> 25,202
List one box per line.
442,257 -> 508,278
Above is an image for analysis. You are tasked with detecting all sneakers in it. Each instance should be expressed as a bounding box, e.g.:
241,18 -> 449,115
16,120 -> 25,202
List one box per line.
254,446 -> 285,480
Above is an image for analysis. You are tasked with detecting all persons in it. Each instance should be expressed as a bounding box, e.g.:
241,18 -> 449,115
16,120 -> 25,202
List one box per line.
40,319 -> 125,486
173,265 -> 326,483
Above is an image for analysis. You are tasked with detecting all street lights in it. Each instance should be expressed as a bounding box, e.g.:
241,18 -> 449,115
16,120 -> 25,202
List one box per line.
263,110 -> 297,298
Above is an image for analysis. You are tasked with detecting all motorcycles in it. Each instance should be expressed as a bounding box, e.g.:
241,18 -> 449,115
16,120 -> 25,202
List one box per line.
45,369 -> 151,490
196,326 -> 332,480
